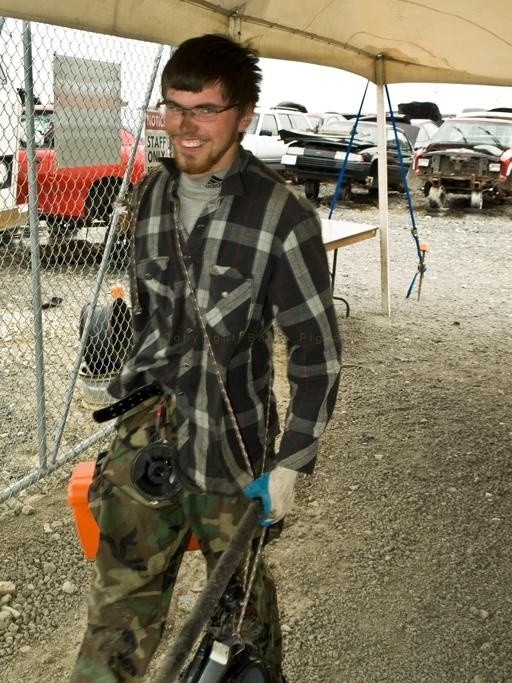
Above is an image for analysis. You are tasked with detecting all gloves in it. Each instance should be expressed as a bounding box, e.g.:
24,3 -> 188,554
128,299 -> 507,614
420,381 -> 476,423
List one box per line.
239,465 -> 299,529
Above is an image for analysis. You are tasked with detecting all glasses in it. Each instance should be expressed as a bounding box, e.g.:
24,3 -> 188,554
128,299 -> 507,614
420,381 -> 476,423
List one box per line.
156,97 -> 239,122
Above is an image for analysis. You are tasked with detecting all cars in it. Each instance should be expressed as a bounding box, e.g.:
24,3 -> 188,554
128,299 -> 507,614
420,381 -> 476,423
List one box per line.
418,117 -> 511,216
0,85 -> 148,229
269,102 -> 512,154
238,107 -> 317,171
277,120 -> 417,202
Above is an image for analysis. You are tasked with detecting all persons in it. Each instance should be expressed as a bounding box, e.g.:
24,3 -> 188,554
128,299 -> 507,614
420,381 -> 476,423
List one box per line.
74,33 -> 343,683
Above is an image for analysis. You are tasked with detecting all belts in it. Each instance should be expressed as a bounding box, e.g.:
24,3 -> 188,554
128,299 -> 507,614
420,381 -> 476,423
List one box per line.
91,381 -> 163,424
